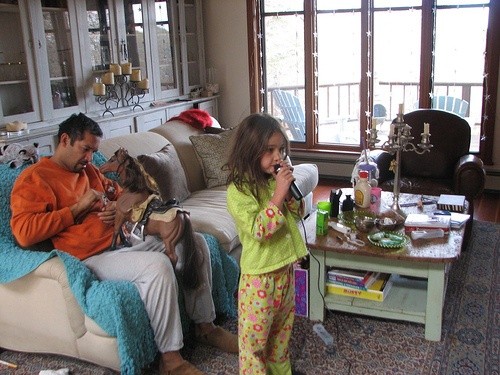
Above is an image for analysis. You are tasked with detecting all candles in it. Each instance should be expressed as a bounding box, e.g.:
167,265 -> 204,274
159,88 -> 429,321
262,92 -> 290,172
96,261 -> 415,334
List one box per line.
130,68 -> 141,82
93,83 -> 105,95
423,122 -> 429,133
120,63 -> 132,74
372,119 -> 377,129
110,63 -> 122,75
398,104 -> 404,114
136,78 -> 149,89
102,72 -> 114,84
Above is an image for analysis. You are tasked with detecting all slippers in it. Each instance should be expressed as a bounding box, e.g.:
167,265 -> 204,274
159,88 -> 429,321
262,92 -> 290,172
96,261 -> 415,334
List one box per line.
159,360 -> 210,375
194,325 -> 240,352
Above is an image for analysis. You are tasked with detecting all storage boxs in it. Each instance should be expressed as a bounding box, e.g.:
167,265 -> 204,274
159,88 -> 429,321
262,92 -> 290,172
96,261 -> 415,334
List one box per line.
293,265 -> 311,316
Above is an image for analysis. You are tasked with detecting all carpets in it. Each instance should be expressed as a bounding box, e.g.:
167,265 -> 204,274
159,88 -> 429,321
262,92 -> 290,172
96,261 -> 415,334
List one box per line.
0,221 -> 500,375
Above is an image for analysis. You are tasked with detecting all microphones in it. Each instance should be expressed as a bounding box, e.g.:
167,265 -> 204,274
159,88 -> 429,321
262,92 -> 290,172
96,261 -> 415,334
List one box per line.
274,163 -> 304,201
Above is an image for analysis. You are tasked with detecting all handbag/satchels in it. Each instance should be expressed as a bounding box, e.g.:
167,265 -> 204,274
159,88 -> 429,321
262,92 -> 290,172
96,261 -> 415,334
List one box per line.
110,192 -> 201,290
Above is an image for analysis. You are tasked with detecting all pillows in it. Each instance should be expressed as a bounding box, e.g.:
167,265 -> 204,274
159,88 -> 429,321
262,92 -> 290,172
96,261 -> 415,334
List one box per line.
189,124 -> 233,189
136,143 -> 192,203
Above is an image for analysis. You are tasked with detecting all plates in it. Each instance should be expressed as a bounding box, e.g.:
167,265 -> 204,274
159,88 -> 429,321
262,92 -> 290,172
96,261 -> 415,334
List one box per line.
338,209 -> 376,224
367,230 -> 411,248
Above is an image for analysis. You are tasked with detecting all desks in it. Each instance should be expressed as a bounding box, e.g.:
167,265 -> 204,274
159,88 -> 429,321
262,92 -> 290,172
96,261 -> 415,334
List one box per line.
304,188 -> 471,342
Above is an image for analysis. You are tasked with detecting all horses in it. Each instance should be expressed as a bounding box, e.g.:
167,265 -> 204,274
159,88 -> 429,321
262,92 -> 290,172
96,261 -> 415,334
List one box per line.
99,146 -> 205,288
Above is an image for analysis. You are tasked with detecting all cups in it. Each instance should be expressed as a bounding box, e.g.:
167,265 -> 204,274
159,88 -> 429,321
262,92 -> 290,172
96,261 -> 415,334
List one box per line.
370,187 -> 382,214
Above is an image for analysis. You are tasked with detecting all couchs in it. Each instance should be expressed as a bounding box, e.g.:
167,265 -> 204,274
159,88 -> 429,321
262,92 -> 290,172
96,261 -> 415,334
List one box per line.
0,119 -> 318,373
350,108 -> 484,225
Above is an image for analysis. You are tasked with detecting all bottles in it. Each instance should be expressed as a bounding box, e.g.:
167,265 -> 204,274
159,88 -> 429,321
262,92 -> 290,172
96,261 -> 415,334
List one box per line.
354,170 -> 372,208
341,195 -> 353,211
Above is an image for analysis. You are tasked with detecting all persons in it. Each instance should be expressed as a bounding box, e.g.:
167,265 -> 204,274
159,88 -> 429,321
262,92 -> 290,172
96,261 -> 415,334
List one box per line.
223,113 -> 309,374
10,112 -> 243,375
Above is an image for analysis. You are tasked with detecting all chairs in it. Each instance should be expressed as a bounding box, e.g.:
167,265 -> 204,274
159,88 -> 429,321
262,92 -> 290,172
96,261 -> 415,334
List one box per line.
270,90 -> 306,142
434,96 -> 468,119
373,104 -> 387,126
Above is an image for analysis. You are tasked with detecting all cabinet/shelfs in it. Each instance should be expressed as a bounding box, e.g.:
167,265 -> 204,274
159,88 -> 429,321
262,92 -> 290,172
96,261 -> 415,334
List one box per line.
0,0 -> 219,156
